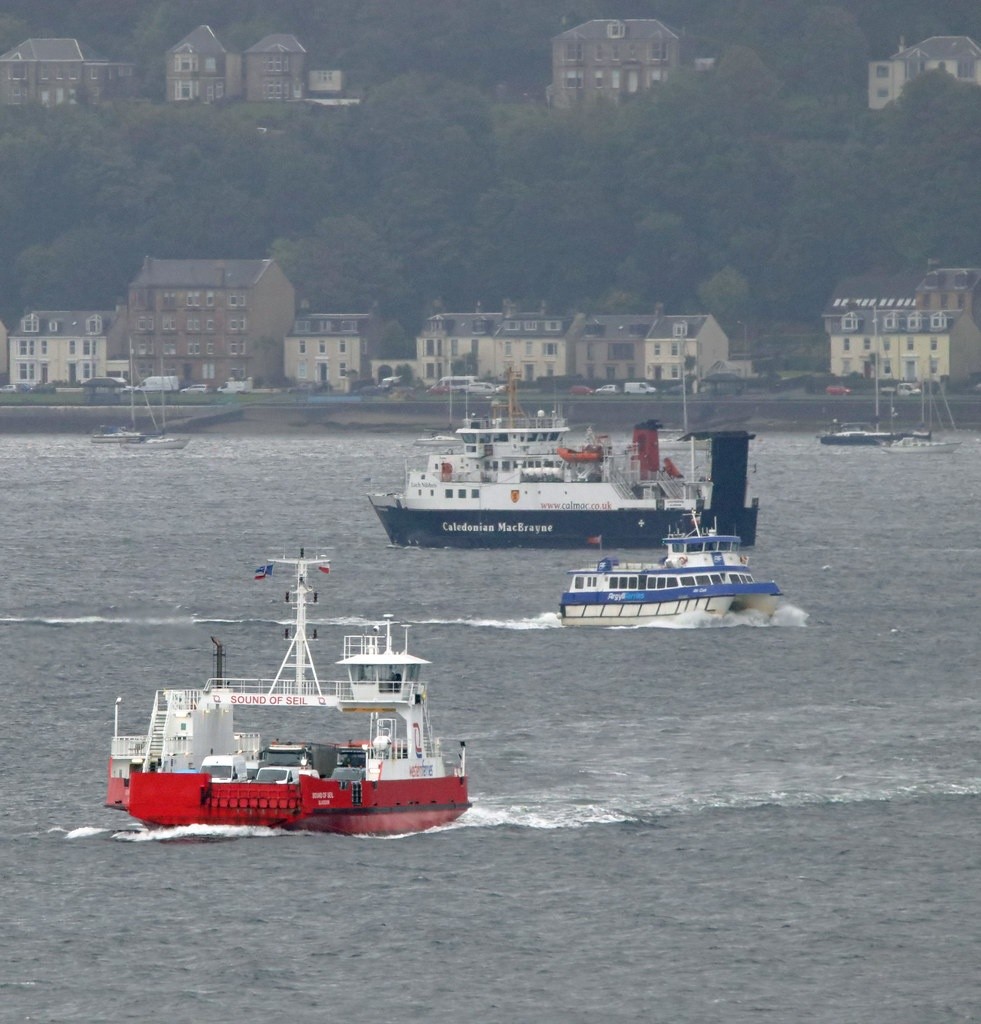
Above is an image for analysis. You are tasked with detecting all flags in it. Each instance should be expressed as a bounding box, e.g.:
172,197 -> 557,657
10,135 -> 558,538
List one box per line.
691,516 -> 701,524
318,564 -> 330,575
587,535 -> 602,544
255,564 -> 273,579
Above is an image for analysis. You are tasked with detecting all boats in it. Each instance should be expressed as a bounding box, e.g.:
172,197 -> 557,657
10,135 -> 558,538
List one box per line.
365,332 -> 760,550
555,446 -> 605,463
556,507 -> 784,628
104,535 -> 472,838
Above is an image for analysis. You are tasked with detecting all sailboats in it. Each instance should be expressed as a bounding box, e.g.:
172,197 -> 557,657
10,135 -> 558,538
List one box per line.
818,304 -> 930,445
879,353 -> 964,455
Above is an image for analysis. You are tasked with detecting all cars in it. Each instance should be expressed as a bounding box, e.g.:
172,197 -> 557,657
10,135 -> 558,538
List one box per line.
0,384 -> 18,394
567,385 -> 594,397
895,383 -> 923,397
825,384 -> 853,396
468,381 -> 495,394
180,384 -> 210,394
330,766 -> 366,781
250,765 -> 300,785
27,383 -> 56,393
595,384 -> 621,394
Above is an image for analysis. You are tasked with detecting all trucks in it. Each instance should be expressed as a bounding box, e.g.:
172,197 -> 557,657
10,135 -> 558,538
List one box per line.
624,382 -> 657,395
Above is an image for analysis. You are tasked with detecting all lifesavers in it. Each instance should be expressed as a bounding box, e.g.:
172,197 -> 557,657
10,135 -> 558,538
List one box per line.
679,557 -> 686,565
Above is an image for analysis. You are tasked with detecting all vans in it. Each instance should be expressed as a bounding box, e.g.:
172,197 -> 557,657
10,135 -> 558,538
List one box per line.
198,754 -> 248,783
428,375 -> 479,393
135,376 -> 180,393
217,380 -> 252,396
